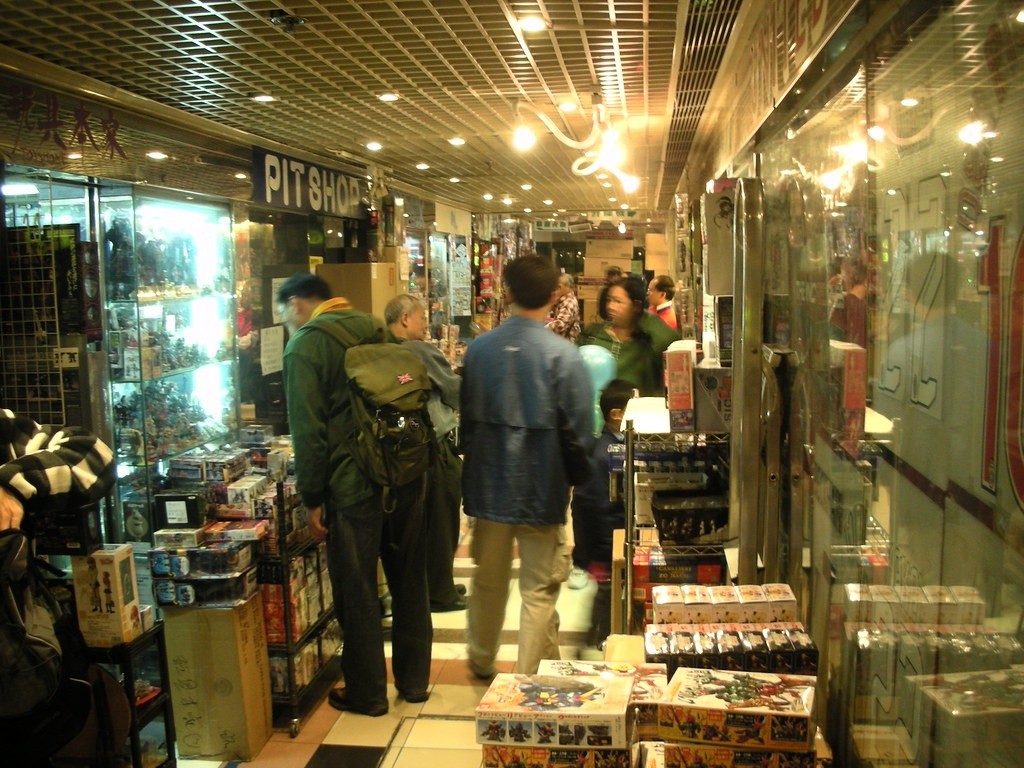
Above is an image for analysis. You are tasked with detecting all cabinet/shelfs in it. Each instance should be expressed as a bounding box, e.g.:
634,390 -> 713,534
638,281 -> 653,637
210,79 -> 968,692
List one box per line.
36,196 -> 347,768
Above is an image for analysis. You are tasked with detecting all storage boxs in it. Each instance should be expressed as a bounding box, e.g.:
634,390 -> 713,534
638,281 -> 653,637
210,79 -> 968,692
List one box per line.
801,340 -> 1024,768
571,177 -> 739,368
35,340 -> 833,768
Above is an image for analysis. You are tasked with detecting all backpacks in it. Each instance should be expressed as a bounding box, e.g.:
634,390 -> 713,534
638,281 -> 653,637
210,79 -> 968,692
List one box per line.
295,314 -> 441,515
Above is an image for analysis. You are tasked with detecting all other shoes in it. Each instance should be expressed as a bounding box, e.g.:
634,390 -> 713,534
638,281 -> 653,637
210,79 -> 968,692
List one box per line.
454,584 -> 466,596
466,658 -> 497,678
329,687 -> 390,717
430,595 -> 472,613
398,689 -> 429,702
566,568 -> 589,590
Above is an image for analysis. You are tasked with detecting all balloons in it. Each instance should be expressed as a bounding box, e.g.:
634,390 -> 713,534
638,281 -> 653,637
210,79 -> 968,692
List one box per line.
580,345 -> 616,392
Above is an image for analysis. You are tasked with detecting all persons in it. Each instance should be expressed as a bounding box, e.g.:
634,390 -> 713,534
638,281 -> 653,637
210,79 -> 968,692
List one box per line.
566,278 -> 661,590
545,274 -> 581,342
385,294 -> 468,613
871,253 -> 1003,585
608,267 -> 643,282
647,275 -> 678,330
588,378 -> 639,650
460,255 -> 594,680
0,407 -> 115,768
829,266 -> 870,349
273,273 -> 434,716
